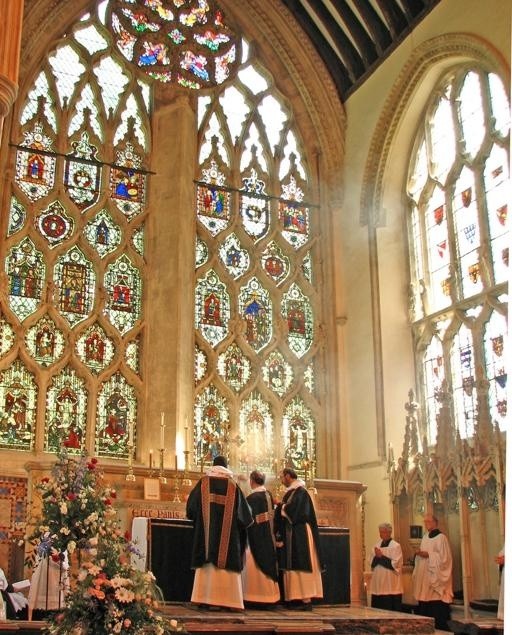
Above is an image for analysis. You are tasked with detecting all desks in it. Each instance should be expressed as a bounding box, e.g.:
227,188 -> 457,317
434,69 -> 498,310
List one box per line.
132,515 -> 350,609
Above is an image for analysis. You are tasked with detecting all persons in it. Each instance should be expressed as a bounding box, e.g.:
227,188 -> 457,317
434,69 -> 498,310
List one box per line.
370,523 -> 404,611
28,528 -> 70,620
412,514 -> 455,631
495,545 -> 504,621
0,568 -> 28,622
275,468 -> 324,611
242,471 -> 281,612
186,455 -> 254,612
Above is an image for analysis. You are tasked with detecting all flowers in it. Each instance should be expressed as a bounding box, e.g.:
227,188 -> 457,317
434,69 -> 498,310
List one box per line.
14,449 -> 187,635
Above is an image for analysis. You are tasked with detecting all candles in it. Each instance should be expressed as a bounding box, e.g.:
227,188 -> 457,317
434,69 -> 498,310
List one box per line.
175,455 -> 177,473
150,453 -> 151,472
129,421 -> 133,447
184,416 -> 187,428
159,412 -> 165,449
